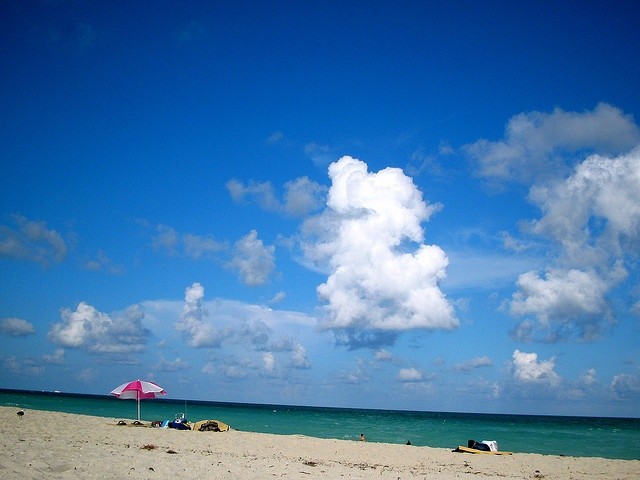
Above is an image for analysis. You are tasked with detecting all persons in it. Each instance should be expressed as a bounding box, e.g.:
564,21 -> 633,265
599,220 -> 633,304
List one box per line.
360,433 -> 365,441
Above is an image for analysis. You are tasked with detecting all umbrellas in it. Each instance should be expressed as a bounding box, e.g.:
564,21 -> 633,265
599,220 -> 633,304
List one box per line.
110,380 -> 167,422
